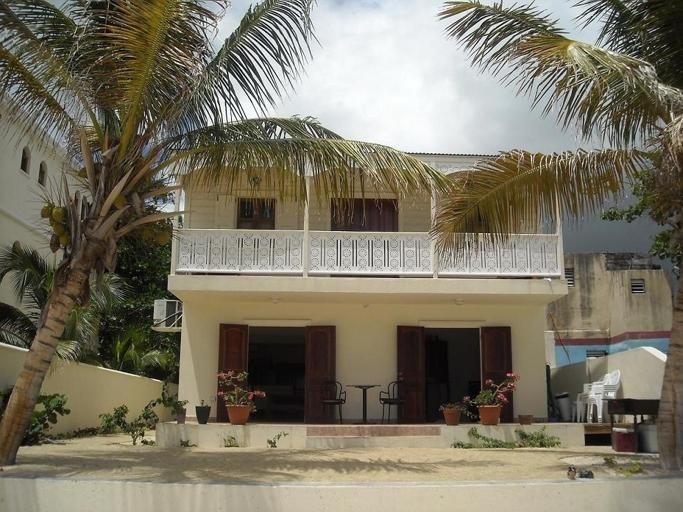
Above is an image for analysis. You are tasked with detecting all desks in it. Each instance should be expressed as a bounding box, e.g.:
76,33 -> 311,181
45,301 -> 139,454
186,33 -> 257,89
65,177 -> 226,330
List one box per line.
346,385 -> 380,424
601,399 -> 659,431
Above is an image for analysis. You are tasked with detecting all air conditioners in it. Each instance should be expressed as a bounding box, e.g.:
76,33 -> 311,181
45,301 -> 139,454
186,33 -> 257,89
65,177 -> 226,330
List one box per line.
154,300 -> 178,327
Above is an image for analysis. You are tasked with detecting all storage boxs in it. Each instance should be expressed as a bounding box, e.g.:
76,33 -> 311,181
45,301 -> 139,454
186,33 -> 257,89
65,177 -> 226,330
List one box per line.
612,428 -> 639,452
639,425 -> 658,452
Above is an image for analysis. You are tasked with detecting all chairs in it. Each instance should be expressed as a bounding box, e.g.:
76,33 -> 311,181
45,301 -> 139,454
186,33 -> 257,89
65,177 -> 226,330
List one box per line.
319,381 -> 346,424
379,381 -> 404,423
572,370 -> 620,423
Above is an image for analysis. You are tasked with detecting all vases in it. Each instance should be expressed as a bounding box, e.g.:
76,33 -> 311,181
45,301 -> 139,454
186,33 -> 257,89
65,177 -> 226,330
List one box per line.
225,405 -> 250,425
518,415 -> 534,425
476,406 -> 503,425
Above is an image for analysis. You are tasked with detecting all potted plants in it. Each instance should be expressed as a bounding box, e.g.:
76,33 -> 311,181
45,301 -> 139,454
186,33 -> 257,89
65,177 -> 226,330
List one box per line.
438,401 -> 477,425
196,400 -> 211,424
173,400 -> 189,423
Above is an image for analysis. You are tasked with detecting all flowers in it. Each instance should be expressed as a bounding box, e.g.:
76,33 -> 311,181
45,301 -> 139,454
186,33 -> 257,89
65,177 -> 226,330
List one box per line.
217,371 -> 266,412
464,373 -> 520,406
